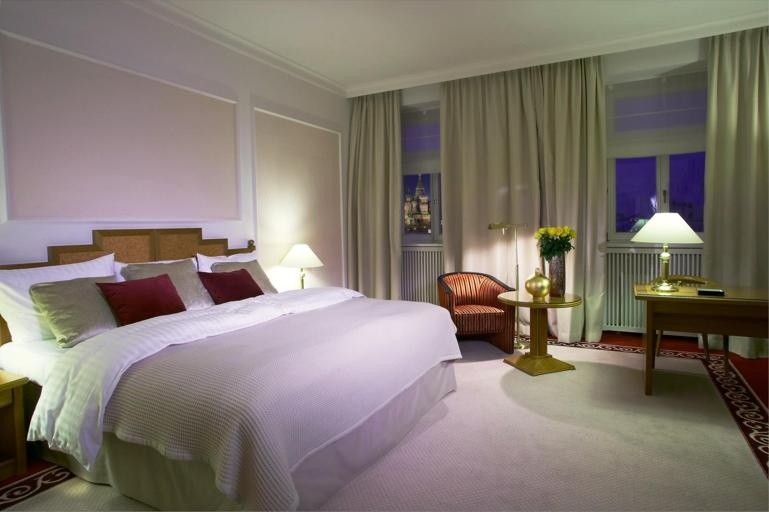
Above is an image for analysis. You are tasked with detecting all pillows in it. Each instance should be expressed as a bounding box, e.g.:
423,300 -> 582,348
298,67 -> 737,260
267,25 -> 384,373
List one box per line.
0,251 -> 284,349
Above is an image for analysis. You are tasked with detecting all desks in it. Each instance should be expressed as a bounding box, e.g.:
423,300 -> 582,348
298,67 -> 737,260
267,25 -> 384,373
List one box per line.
632,280 -> 768,396
497,285 -> 585,378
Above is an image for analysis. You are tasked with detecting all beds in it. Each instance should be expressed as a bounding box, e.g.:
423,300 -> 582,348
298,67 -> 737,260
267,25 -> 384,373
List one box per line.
0,226 -> 463,507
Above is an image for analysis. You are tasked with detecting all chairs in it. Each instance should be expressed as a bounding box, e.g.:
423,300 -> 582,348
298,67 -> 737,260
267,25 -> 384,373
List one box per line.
648,272 -> 735,378
431,270 -> 520,357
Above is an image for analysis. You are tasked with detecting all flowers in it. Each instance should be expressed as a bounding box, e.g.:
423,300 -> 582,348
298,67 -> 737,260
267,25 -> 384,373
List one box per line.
533,223 -> 578,260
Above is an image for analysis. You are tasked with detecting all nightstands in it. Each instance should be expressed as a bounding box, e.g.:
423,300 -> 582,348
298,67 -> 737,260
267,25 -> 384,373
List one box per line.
0,369 -> 32,482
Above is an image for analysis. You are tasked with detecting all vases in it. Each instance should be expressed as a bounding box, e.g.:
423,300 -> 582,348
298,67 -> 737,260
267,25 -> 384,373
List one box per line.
545,246 -> 566,299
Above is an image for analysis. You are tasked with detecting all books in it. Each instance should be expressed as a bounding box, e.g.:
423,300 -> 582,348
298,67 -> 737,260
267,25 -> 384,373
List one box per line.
696,285 -> 726,297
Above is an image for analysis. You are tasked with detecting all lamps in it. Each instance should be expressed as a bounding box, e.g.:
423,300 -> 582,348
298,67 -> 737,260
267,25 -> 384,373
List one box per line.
280,243 -> 326,289
629,209 -> 707,293
